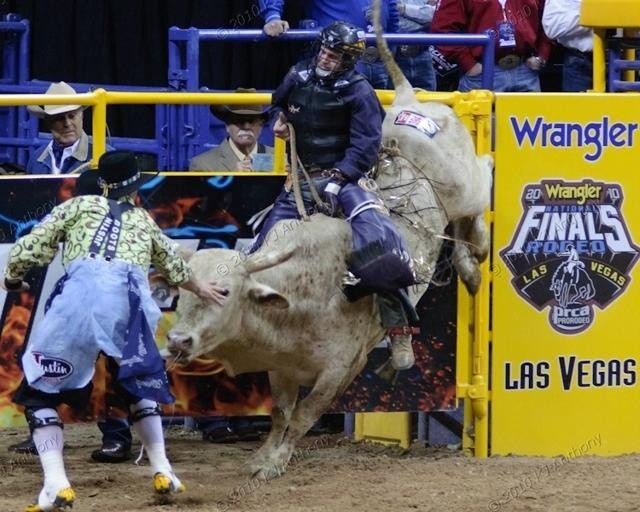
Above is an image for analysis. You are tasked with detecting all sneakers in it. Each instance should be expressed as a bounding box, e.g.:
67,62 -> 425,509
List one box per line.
8,434 -> 39,456
386,330 -> 416,372
23,486 -> 79,512
90,421 -> 132,464
150,471 -> 188,497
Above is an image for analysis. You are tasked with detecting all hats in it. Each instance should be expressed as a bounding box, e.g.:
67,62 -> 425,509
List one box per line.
24,78 -> 95,118
208,86 -> 275,123
75,148 -> 162,201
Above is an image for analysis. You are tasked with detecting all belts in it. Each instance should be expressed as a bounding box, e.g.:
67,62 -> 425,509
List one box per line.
496,54 -> 536,71
397,44 -> 429,56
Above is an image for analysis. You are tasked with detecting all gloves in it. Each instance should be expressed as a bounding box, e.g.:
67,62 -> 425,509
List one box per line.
316,180 -> 343,220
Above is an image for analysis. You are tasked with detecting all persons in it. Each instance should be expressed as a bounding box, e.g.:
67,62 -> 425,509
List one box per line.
558,245 -> 586,273
189,88 -> 289,172
246,21 -> 415,370
26,81 -> 115,175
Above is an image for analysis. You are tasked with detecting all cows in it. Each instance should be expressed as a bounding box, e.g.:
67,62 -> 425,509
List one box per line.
164,0 -> 495,483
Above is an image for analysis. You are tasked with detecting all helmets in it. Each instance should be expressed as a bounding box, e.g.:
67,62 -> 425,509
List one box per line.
304,21 -> 367,85
